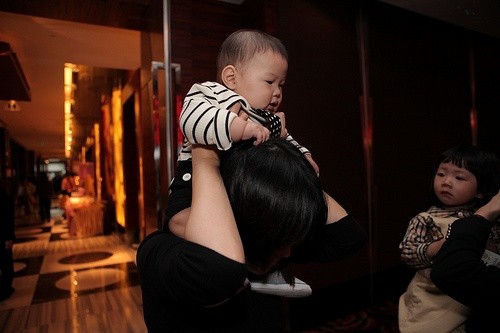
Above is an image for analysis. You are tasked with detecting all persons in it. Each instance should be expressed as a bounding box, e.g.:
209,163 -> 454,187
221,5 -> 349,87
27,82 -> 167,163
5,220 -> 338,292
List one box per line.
168,31 -> 315,299
399,189 -> 500,333
0,174 -> 16,301
21,168 -> 75,226
401,146 -> 500,282
136,103 -> 368,333
